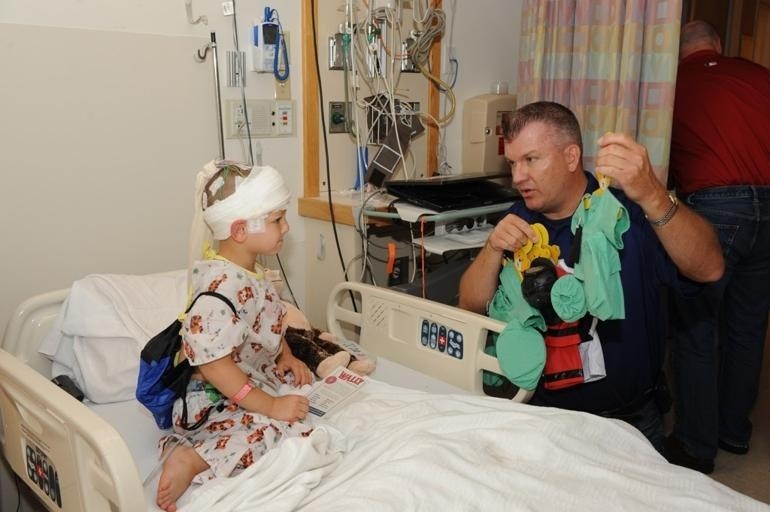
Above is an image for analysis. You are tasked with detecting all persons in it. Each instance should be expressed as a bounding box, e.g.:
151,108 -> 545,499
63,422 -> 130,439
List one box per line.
458,98 -> 726,459
665,20 -> 769,474
157,159 -> 313,512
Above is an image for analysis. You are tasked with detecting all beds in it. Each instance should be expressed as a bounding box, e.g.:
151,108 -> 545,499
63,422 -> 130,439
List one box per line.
2,281 -> 770,512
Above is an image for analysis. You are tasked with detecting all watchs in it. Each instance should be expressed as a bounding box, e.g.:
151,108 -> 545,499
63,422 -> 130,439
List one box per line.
645,189 -> 679,228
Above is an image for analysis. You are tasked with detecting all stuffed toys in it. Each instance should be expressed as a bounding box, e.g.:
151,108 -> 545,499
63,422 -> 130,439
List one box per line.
261,268 -> 376,378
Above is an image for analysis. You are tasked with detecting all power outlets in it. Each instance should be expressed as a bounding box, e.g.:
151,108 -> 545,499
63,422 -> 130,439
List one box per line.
330,101 -> 352,133
233,103 -> 252,135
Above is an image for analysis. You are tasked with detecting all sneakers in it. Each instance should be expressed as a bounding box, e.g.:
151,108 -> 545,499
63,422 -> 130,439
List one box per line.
651,434 -> 714,475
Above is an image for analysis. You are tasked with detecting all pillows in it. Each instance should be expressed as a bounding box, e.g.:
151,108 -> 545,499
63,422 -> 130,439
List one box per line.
55,271 -> 192,402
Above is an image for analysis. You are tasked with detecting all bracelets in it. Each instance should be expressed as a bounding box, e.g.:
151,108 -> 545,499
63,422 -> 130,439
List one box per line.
232,378 -> 257,404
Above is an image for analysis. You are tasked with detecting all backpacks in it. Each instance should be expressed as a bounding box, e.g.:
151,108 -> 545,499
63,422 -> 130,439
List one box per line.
134,291 -> 238,429
